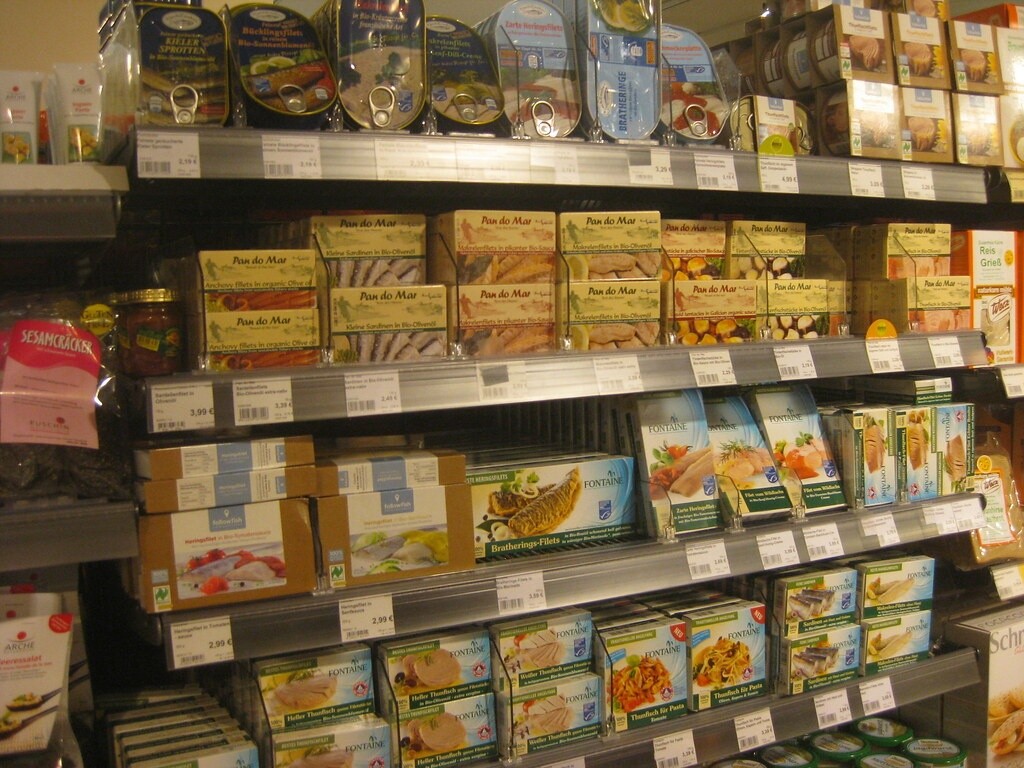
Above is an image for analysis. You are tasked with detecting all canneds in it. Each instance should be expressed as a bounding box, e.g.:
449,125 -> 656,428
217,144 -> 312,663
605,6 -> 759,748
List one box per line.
707,716 -> 970,768
98,0 -> 815,158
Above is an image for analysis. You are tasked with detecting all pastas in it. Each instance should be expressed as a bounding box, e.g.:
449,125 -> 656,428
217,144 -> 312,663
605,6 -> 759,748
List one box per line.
606,654 -> 673,712
696,637 -> 753,689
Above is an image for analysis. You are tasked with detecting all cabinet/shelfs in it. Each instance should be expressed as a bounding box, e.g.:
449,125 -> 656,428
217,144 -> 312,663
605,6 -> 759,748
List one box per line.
1,130 -> 1023,768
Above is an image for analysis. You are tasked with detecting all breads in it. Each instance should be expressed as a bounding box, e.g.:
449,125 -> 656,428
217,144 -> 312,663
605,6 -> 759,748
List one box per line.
936,454 -> 1024,570
